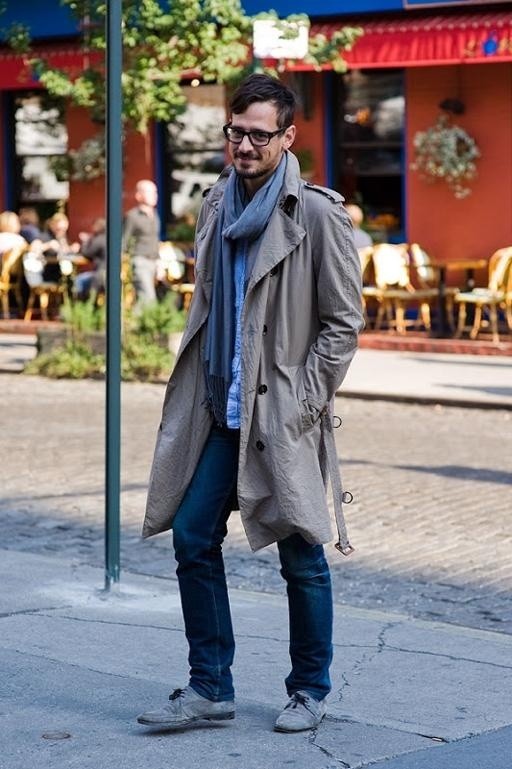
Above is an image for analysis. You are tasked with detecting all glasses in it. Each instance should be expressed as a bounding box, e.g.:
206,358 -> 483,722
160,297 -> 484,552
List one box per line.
222,122 -> 286,147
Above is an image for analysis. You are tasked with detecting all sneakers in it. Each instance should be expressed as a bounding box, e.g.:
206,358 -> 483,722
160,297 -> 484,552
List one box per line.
137,688 -> 235,725
274,689 -> 326,733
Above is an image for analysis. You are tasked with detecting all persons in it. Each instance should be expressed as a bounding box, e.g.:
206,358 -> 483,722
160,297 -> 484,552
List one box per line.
136,73 -> 364,734
343,204 -> 375,250
1,207 -> 193,322
122,180 -> 163,318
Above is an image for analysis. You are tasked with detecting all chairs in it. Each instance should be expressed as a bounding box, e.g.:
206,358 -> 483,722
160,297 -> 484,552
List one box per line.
359,244 -> 512,348
